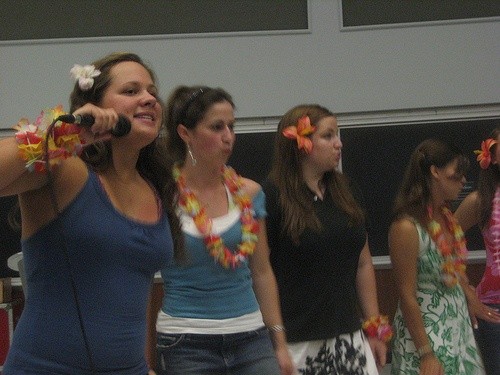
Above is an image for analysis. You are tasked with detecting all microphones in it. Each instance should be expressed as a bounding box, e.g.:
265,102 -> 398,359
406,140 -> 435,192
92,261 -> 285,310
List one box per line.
59,112 -> 131,137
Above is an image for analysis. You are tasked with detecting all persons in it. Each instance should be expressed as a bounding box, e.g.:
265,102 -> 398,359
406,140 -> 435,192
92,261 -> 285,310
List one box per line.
0,52 -> 174,375
151,86 -> 296,375
391,136 -> 500,375
266,103 -> 392,375
454,127 -> 500,375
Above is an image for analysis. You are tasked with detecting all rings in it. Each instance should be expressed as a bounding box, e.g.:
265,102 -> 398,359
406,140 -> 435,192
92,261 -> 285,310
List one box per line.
487,312 -> 492,316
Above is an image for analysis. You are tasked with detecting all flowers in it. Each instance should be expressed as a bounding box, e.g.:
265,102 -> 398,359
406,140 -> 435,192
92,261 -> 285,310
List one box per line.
281,116 -> 317,153
70,61 -> 100,89
474,138 -> 497,169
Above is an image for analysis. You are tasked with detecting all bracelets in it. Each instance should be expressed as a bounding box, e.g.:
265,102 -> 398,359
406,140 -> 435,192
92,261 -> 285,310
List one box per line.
16,104 -> 84,175
418,343 -> 432,357
363,314 -> 395,342
269,325 -> 286,336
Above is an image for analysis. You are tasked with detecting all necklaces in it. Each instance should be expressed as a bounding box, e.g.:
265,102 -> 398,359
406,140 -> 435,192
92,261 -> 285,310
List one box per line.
170,162 -> 260,271
419,201 -> 467,288
